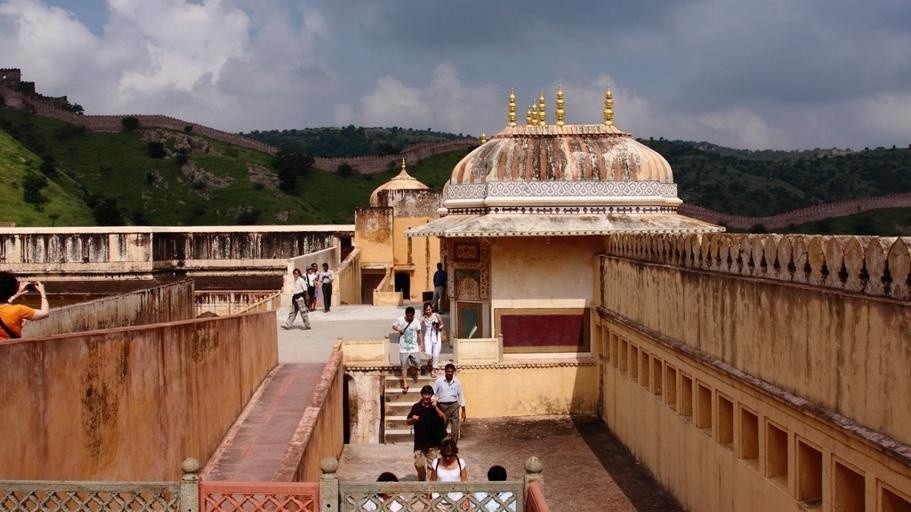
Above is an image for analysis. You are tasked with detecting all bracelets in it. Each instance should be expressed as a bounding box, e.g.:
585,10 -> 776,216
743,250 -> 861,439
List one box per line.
12,293 -> 18,299
42,295 -> 48,299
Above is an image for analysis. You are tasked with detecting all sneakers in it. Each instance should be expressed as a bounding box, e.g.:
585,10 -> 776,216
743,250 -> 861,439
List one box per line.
280,307 -> 331,330
403,369 -> 437,395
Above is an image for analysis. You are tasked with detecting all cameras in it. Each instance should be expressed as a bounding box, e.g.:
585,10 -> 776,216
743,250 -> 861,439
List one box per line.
27,283 -> 38,293
432,321 -> 439,329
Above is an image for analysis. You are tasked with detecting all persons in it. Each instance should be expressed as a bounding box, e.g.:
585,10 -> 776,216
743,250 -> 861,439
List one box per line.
304,265 -> 318,309
363,472 -> 406,511
281,268 -> 312,330
432,263 -> 448,314
0,271 -> 51,341
408,385 -> 448,481
393,306 -> 425,394
311,263 -> 321,310
471,464 -> 516,511
320,262 -> 335,312
424,439 -> 472,511
418,300 -> 445,378
434,363 -> 466,445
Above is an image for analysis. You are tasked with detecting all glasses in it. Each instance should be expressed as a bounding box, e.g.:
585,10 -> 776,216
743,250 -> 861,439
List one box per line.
440,451 -> 455,458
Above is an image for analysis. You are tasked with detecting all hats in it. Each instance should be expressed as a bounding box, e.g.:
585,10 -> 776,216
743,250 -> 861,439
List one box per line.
305,266 -> 313,271
